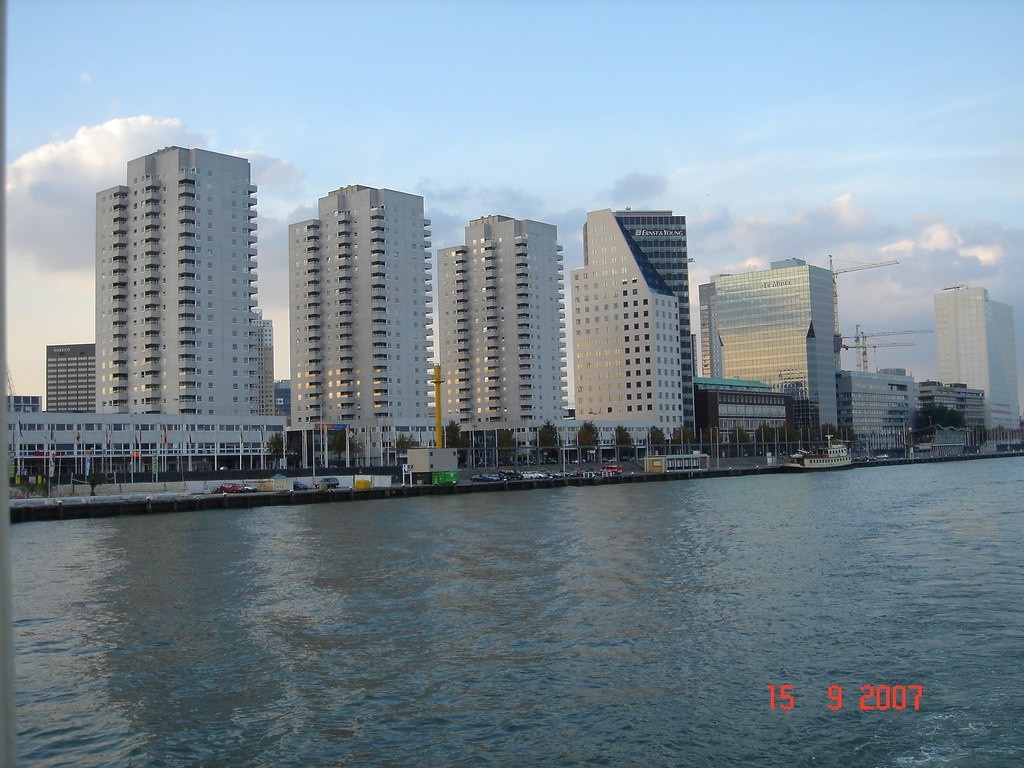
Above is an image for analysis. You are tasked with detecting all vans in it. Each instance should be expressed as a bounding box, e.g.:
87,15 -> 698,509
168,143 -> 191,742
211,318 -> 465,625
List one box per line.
600,463 -> 623,475
220,466 -> 228,470
218,482 -> 244,493
499,470 -> 524,480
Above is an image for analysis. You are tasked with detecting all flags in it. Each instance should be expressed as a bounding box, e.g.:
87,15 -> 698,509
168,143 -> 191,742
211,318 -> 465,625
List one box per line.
152,451 -> 158,474
49,451 -> 56,477
19,420 -> 24,437
51,428 -> 56,442
85,451 -> 91,477
10,452 -> 14,478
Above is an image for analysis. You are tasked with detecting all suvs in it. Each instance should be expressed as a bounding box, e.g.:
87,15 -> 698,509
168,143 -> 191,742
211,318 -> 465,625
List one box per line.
315,478 -> 340,490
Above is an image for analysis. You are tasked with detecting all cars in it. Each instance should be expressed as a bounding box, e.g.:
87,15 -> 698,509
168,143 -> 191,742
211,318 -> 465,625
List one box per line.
293,481 -> 308,491
601,455 -> 644,463
877,454 -> 889,459
521,470 -> 584,480
490,473 -> 511,481
240,484 -> 258,492
471,473 -> 500,482
852,455 -> 876,462
458,457 -> 587,467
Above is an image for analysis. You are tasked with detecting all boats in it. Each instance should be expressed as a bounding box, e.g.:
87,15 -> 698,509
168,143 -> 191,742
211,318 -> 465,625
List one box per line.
777,435 -> 853,473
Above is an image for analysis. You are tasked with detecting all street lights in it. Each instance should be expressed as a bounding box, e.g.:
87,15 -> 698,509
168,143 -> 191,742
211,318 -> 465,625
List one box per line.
442,425 -> 446,448
715,426 -> 719,470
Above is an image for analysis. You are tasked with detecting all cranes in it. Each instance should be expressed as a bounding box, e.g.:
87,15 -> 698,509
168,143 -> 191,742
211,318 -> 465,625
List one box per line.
840,325 -> 934,371
825,254 -> 900,370
